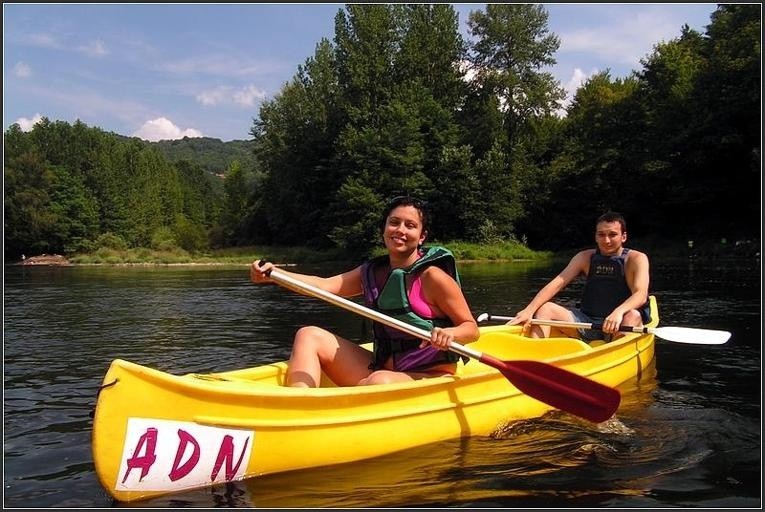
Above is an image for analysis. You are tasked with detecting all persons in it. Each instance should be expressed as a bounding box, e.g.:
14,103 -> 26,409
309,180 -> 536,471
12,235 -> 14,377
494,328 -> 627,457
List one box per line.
250,196 -> 480,388
505,212 -> 650,343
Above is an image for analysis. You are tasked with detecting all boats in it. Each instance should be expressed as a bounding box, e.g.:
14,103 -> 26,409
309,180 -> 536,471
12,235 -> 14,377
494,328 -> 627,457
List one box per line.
91,295 -> 659,503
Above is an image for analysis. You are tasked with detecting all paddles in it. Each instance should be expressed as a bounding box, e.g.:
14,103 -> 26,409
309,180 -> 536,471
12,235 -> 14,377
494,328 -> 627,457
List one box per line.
477,312 -> 732,346
256,258 -> 623,423
